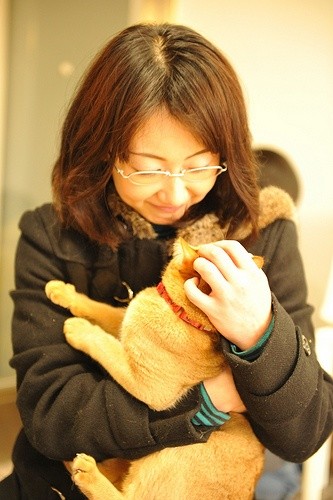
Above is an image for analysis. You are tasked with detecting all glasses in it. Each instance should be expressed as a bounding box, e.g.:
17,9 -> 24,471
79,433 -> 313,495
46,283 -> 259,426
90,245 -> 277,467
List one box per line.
113,162 -> 227,185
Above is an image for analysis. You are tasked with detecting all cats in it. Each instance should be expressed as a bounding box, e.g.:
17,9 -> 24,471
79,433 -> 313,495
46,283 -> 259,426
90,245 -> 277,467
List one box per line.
44,234 -> 266,500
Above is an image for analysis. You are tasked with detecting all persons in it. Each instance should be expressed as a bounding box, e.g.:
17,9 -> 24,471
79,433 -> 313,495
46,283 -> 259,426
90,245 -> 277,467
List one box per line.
0,24 -> 332,500
